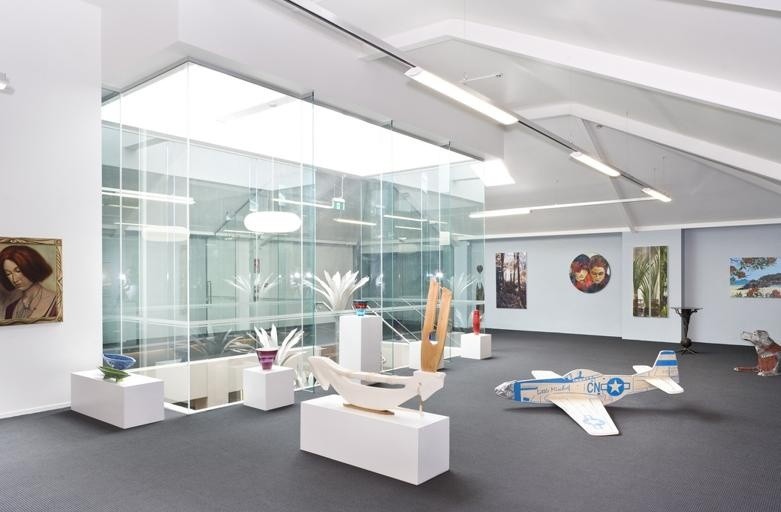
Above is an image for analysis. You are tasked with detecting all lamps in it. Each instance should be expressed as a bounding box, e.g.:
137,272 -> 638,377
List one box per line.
242,211 -> 304,235
394,225 -> 423,230
332,216 -> 380,229
639,185 -> 676,205
565,148 -> 625,180
468,206 -> 535,219
383,213 -> 428,223
403,65 -> 523,128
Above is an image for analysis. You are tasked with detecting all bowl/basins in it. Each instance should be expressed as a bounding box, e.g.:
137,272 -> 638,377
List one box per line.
100,366 -> 130,382
105,352 -> 135,369
257,348 -> 278,370
352,300 -> 368,316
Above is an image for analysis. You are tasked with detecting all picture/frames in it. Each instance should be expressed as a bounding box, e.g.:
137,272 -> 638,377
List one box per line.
1,238 -> 63,325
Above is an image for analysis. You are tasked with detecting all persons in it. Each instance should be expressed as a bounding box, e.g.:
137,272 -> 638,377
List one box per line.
0,246 -> 58,320
570,255 -> 592,293
588,254 -> 610,293
